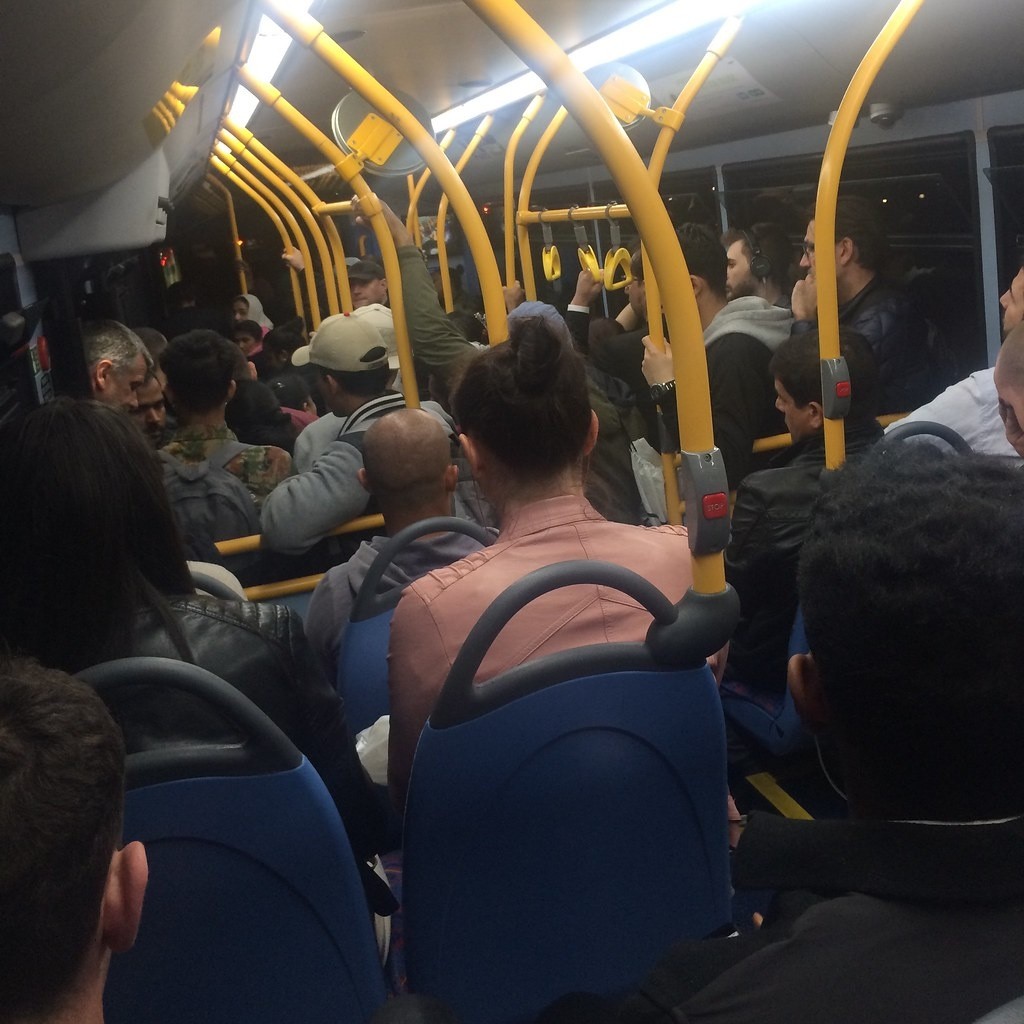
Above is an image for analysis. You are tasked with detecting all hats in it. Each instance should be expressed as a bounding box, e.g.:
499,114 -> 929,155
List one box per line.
355,302 -> 401,370
224,378 -> 281,429
291,310 -> 390,372
347,259 -> 385,282
333,257 -> 360,276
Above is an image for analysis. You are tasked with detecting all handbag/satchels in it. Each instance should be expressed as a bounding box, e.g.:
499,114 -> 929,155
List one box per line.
627,436 -> 686,529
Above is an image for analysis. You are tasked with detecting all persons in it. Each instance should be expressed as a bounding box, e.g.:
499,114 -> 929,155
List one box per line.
0,193 -> 1023,853
535,443 -> 1023,1024
0,660 -> 148,1024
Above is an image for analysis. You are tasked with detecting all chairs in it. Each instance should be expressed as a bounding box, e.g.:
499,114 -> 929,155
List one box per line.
70,415 -> 1023,1024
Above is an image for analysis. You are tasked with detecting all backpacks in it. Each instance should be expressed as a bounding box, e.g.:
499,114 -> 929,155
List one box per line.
156,441 -> 261,588
